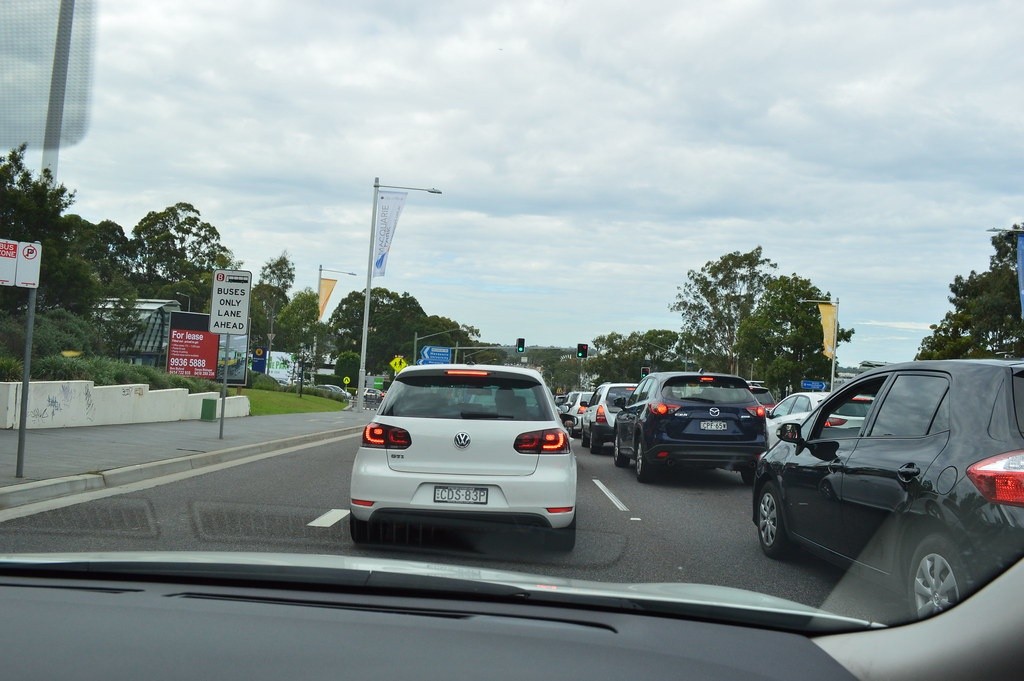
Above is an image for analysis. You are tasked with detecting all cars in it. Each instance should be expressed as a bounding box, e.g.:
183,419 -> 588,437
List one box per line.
315,385 -> 352,400
355,387 -> 385,401
752,358 -> 1023,622
277,377 -> 311,388
554,392 -> 595,439
765,391 -> 876,449
469,387 -> 538,407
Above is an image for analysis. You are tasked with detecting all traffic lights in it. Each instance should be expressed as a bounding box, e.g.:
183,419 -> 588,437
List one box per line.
577,344 -> 588,358
640,367 -> 650,378
517,338 -> 525,352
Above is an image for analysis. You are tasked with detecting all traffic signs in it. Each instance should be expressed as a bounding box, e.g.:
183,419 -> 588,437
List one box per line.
800,379 -> 826,391
420,345 -> 451,359
417,358 -> 451,364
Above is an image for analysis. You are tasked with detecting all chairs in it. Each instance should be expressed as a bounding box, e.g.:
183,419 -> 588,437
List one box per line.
500,396 -> 528,419
662,383 -> 680,399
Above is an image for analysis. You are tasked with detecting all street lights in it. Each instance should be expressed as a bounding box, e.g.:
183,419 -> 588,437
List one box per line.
413,327 -> 464,365
356,178 -> 443,413
311,264 -> 357,385
798,297 -> 840,391
175,291 -> 190,312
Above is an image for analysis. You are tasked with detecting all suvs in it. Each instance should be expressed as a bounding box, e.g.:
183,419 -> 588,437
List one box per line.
349,364 -> 580,553
747,386 -> 777,411
612,371 -> 769,486
579,382 -> 640,454
